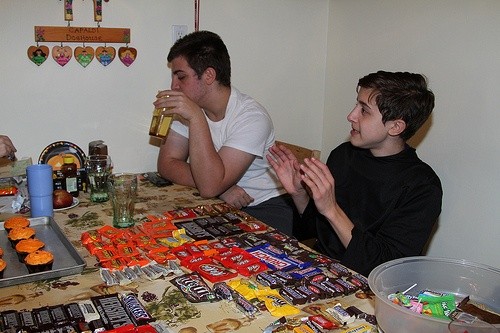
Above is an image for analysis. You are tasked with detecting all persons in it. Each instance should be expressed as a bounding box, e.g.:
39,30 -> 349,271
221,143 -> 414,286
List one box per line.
158,31 -> 293,238
266,71 -> 443,278
0,135 -> 17,160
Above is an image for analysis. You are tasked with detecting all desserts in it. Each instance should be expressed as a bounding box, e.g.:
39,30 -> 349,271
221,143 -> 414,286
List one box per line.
0,216 -> 54,280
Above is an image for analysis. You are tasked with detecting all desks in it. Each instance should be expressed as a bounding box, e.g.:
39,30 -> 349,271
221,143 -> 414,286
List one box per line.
0,172 -> 383,333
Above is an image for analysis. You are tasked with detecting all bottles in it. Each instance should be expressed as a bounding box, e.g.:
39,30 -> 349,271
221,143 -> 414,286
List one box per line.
87,155 -> 112,202
61,156 -> 80,197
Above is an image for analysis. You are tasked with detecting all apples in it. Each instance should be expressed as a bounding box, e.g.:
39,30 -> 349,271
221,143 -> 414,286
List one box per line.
52,190 -> 74,209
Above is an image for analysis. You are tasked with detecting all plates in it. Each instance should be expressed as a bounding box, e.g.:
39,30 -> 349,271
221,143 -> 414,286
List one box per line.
38,141 -> 86,171
53,197 -> 79,212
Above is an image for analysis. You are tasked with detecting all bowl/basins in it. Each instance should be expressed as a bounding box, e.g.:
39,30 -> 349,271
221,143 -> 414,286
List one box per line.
367,256 -> 500,333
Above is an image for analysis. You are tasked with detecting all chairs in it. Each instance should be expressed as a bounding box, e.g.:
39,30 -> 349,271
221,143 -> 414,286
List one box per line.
274,140 -> 322,169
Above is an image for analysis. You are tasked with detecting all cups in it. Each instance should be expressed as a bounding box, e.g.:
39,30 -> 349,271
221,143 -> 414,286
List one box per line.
107,173 -> 138,227
149,92 -> 176,140
26,164 -> 54,218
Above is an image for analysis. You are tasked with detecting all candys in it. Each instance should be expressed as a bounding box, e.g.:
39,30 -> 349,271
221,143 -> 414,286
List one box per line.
0,291 -> 157,333
82,203 -> 381,333
387,283 -> 500,327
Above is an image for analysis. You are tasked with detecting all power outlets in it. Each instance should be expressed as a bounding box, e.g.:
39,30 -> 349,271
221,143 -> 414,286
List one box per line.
172,25 -> 187,43
